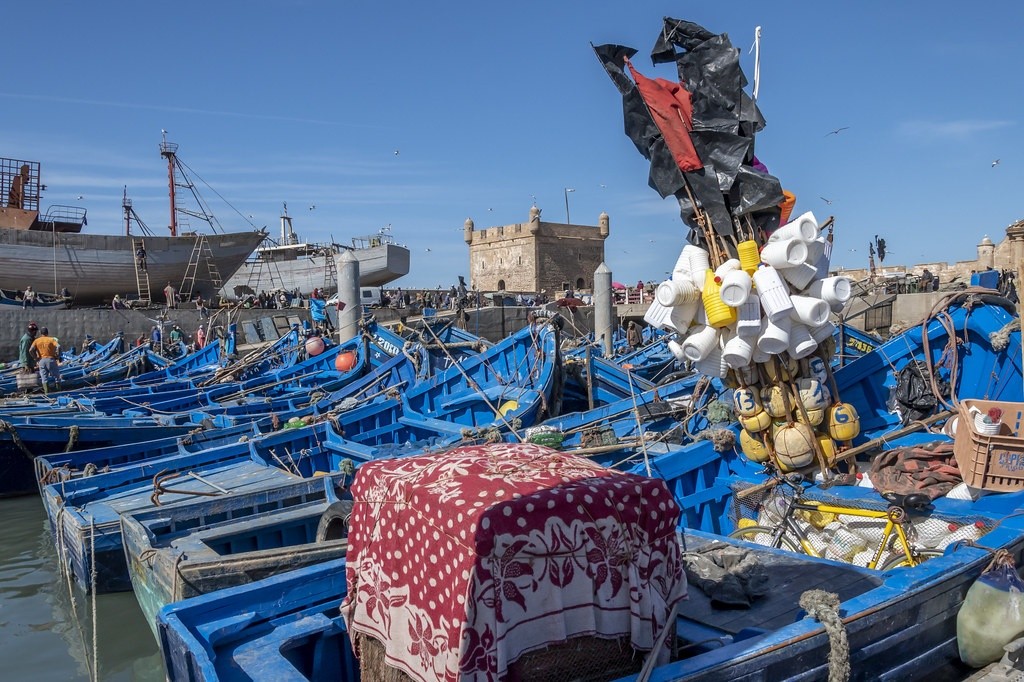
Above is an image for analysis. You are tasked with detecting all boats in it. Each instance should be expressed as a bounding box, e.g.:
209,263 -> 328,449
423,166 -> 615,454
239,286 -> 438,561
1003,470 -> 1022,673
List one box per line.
156,270 -> 1023,682
218,200 -> 411,306
0,129 -> 270,307
0,318 -> 884,651
0,288 -> 74,310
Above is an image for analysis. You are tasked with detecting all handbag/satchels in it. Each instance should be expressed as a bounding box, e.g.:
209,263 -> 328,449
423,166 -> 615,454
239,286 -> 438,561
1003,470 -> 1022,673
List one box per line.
16,370 -> 38,388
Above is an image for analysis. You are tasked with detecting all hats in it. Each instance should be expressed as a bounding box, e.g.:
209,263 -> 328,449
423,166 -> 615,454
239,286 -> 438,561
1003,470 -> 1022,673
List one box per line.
28,324 -> 38,330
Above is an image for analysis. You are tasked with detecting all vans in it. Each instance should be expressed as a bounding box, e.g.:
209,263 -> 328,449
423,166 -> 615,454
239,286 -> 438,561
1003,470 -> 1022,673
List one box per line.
359,287 -> 382,309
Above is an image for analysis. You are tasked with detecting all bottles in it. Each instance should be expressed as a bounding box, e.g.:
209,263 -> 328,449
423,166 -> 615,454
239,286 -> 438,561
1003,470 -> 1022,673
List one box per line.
788,294 -> 831,327
720,269 -> 752,307
753,264 -> 793,322
283,421 -> 306,429
669,324 -> 720,361
808,275 -> 851,306
657,280 -> 697,306
644,298 -> 698,334
701,269 -> 735,327
781,263 -> 818,290
754,497 -> 927,570
695,343 -> 728,379
753,349 -> 772,363
723,328 -> 757,368
907,520 -> 958,548
768,218 -> 817,246
736,294 -> 761,337
786,316 -> 818,360
807,321 -> 835,344
736,240 -> 761,288
805,236 -> 825,265
713,258 -> 740,284
759,239 -> 809,269
935,520 -> 984,551
671,245 -> 709,292
757,309 -> 791,355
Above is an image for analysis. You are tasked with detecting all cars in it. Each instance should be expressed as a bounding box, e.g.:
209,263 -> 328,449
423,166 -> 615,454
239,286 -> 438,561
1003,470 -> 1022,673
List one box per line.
327,293 -> 339,306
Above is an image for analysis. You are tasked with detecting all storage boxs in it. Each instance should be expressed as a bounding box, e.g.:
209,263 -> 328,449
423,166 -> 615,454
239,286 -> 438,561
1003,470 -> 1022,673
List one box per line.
953,398 -> 1024,492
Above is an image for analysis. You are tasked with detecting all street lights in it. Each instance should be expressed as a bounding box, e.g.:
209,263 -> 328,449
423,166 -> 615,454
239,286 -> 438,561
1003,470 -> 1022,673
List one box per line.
564,188 -> 575,224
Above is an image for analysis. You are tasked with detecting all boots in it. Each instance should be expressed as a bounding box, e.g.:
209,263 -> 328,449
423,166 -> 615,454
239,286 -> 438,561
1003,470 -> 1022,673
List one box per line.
55,382 -> 62,392
43,382 -> 48,394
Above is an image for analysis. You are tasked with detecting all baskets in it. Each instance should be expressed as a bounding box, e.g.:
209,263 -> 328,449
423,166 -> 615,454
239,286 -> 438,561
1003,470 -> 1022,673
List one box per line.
954,399 -> 1024,492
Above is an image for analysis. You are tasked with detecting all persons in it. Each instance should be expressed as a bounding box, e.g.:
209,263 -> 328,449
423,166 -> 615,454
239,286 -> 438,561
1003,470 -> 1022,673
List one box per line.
382,287 -> 410,305
312,288 -> 318,298
919,268 -> 933,292
637,281 -> 643,292
112,294 -> 124,309
646,282 -> 653,293
164,282 -> 176,307
196,296 -> 209,320
259,289 -> 266,309
22,286 -> 35,309
18,324 -> 38,374
197,325 -> 205,349
567,288 -> 574,298
416,288 -> 450,308
137,247 -> 146,271
137,332 -> 145,347
29,327 -> 61,394
170,325 -> 179,343
152,326 -> 161,341
450,285 -> 457,310
318,288 -> 323,298
627,321 -> 642,347
